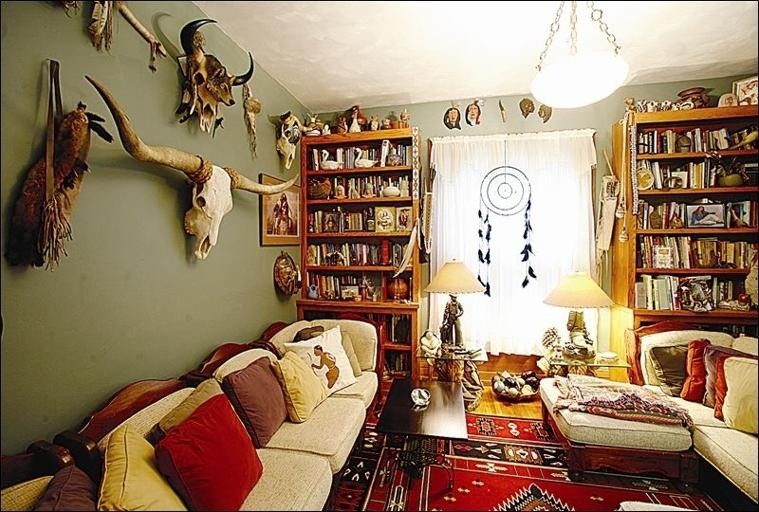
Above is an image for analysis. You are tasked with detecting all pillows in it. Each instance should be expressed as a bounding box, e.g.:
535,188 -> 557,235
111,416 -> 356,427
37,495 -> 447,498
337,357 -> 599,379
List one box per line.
33,325 -> 362,511
643,338 -> 759,438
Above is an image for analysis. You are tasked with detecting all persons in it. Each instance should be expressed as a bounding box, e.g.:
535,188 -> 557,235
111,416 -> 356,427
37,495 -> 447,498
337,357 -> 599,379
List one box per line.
465,103 -> 481,127
311,344 -> 339,388
442,295 -> 464,347
442,108 -> 462,130
273,194 -> 293,233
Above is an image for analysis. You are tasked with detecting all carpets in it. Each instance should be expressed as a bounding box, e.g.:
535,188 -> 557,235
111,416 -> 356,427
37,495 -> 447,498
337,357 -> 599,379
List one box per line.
328,411 -> 727,510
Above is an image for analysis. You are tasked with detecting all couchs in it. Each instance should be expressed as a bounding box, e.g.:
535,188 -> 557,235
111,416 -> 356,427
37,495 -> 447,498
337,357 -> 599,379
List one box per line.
539,373 -> 701,494
625,320 -> 759,512
0,313 -> 386,512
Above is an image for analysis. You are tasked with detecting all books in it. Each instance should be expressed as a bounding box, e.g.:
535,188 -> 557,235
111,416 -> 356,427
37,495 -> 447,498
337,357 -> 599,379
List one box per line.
306,139 -> 412,305
634,128 -> 759,310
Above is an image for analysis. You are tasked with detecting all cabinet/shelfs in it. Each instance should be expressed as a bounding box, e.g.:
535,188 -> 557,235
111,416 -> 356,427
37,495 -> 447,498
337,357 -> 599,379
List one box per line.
610,105 -> 759,366
296,126 -> 421,379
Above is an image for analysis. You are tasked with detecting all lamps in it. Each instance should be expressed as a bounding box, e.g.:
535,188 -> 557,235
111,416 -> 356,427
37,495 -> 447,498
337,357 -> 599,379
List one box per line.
543,270 -> 616,359
531,1 -> 629,110
423,259 -> 487,347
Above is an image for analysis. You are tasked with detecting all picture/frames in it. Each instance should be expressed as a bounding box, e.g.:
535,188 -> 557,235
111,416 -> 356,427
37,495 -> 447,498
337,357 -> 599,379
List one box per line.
731,75 -> 759,106
322,212 -> 340,233
339,283 -> 360,299
259,173 -> 301,246
394,206 -> 413,233
374,205 -> 396,233
685,203 -> 726,228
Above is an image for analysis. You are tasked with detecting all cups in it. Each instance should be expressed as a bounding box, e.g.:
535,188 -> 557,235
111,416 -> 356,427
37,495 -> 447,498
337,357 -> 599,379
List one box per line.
353,295 -> 361,302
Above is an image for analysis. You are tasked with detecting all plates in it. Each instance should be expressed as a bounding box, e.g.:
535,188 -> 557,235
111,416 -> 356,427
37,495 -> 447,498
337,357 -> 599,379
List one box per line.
600,351 -> 618,359
